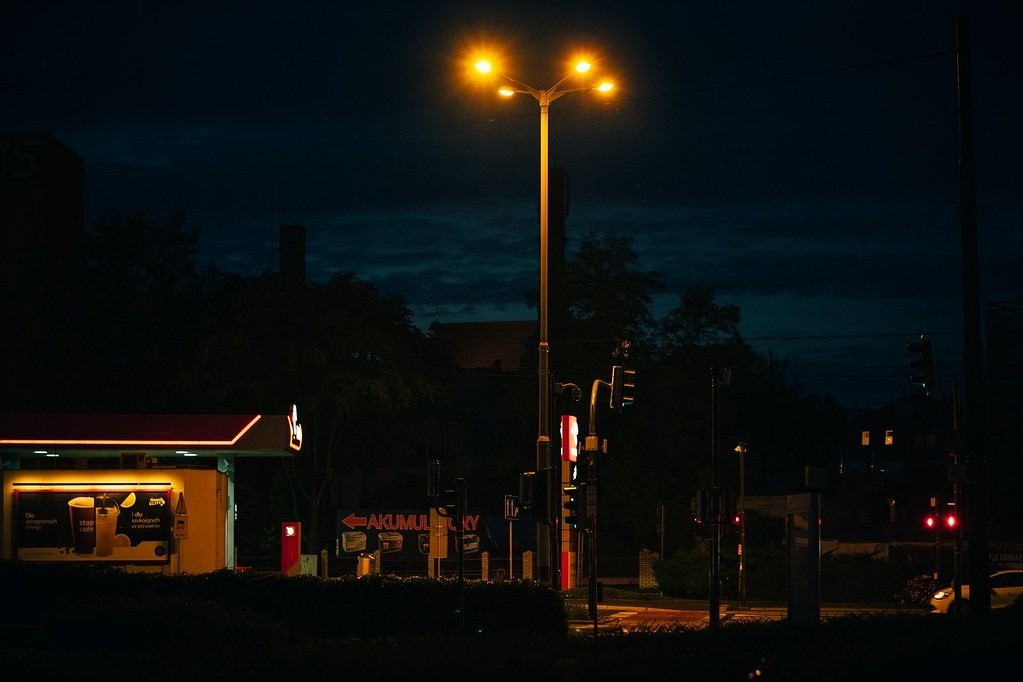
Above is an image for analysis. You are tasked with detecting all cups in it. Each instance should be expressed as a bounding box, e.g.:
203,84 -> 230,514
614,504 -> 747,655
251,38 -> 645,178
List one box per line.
67,497 -> 96,556
95,507 -> 120,557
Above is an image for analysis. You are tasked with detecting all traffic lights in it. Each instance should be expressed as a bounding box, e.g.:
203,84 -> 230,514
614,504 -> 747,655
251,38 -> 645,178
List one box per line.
927,512 -> 935,533
946,511 -> 956,534
609,366 -> 637,409
563,482 -> 586,531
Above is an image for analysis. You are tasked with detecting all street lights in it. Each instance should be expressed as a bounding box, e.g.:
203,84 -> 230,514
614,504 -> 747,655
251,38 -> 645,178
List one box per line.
468,49 -> 617,590
735,441 -> 747,600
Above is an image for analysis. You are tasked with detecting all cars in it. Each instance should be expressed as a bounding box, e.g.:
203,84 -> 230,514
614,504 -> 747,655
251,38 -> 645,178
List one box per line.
928,569 -> 1023,617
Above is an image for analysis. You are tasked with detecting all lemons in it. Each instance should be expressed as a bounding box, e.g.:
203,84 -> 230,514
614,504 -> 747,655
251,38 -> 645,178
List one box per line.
120,493 -> 136,508
114,534 -> 131,548
